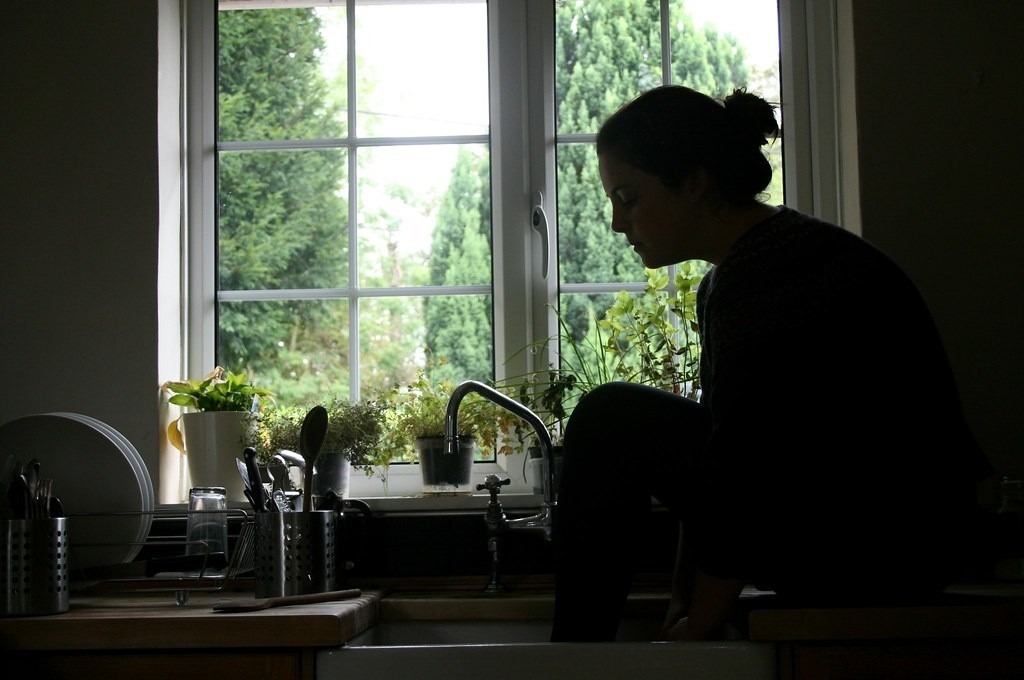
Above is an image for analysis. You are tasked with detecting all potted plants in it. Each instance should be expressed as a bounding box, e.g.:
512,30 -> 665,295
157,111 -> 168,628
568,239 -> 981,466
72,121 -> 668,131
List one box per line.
238,347 -> 576,499
160,366 -> 278,504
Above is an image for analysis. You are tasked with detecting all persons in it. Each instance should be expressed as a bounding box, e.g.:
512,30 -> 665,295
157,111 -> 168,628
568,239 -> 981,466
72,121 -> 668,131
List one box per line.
548,85 -> 979,643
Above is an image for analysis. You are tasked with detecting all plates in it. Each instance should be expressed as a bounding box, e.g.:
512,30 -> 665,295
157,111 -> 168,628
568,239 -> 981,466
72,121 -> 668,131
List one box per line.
0,412 -> 155,562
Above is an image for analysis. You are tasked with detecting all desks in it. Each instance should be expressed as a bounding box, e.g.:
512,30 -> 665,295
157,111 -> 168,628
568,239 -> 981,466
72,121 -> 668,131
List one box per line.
0,590 -> 382,680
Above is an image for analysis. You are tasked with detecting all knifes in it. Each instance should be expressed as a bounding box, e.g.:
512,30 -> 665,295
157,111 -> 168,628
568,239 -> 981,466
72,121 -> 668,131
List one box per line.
236,457 -> 268,500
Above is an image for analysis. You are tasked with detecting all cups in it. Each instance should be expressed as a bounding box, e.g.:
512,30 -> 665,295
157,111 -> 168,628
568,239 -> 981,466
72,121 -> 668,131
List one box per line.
185,487 -> 229,575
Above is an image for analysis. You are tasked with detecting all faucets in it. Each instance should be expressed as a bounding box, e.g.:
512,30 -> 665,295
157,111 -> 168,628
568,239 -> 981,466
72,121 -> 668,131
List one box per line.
445,376 -> 560,595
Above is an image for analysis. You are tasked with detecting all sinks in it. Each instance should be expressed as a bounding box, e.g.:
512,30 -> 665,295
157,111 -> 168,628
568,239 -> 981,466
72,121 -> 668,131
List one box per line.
353,621 -> 556,645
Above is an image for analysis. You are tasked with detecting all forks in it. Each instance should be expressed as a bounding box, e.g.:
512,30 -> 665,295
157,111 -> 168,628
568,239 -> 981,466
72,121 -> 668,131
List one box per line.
32,478 -> 54,519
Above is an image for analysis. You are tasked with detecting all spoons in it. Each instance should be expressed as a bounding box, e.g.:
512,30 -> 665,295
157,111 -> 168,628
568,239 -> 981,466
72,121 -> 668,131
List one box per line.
276,448 -> 318,512
299,406 -> 327,512
10,456 -> 40,519
